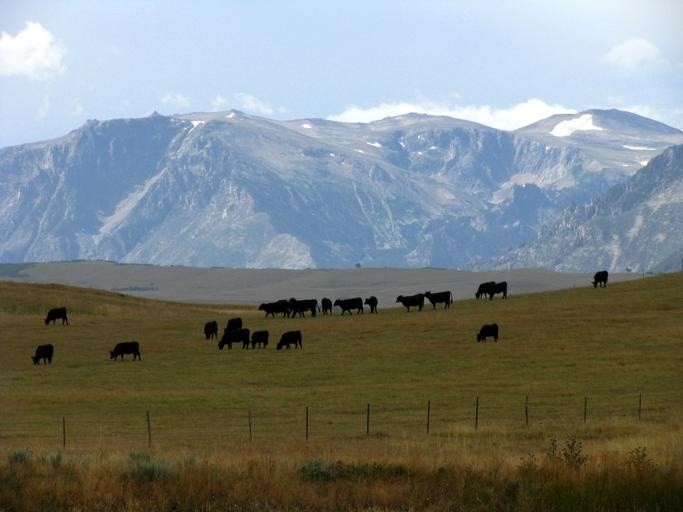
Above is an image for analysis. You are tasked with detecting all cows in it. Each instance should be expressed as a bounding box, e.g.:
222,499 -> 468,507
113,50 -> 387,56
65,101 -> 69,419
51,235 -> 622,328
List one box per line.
591,271 -> 608,288
475,282 -> 507,301
276,330 -> 302,350
477,324 -> 498,344
109,342 -> 141,361
43,307 -> 68,325
218,318 -> 269,350
204,318 -> 216,337
394,290 -> 454,313
258,296 -> 378,318
31,344 -> 53,366
